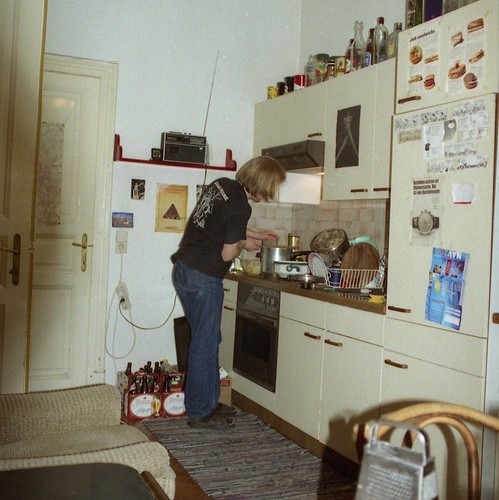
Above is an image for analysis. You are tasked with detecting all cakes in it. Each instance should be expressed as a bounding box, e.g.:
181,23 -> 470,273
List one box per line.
448,63 -> 466,79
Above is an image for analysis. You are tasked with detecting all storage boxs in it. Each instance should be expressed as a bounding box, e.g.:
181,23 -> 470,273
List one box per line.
117,371 -> 232,422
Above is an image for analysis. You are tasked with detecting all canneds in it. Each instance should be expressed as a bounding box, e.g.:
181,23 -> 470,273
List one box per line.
325,56 -> 346,81
267,85 -> 278,99
293,75 -> 310,90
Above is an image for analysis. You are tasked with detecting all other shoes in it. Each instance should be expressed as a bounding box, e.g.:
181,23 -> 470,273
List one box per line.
187,414 -> 237,429
212,403 -> 237,414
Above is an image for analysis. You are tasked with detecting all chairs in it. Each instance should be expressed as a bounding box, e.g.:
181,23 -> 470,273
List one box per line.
0,384 -> 176,500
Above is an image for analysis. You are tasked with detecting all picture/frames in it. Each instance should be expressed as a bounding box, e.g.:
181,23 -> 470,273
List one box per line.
154,183 -> 188,233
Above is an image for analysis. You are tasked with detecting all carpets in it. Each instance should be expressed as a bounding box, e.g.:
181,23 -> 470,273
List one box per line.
144,404 -> 357,500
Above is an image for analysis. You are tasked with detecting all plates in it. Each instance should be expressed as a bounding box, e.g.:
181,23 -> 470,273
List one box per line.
310,228 -> 350,264
307,235 -> 380,284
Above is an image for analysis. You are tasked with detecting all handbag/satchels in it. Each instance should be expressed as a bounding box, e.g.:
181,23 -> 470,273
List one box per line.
354,419 -> 440,500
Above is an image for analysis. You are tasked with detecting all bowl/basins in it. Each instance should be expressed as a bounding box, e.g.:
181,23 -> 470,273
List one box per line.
239,258 -> 262,276
272,260 -> 311,279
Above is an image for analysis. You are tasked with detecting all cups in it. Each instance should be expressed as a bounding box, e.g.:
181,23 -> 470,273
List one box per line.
300,274 -> 312,290
266,74 -> 309,99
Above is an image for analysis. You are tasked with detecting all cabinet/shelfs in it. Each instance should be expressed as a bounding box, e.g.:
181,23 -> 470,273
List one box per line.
382,1 -> 499,500
275,283 -> 385,480
219,272 -> 238,404
321,58 -> 397,201
252,81 -> 324,203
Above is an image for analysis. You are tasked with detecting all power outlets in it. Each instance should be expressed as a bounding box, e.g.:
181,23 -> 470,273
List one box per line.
115,242 -> 127,253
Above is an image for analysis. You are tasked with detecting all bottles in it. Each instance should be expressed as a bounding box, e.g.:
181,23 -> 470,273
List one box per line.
304,16 -> 403,89
126,360 -> 170,396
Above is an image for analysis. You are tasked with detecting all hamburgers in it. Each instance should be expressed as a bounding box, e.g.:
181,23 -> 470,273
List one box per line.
463,73 -> 478,89
424,75 -> 435,89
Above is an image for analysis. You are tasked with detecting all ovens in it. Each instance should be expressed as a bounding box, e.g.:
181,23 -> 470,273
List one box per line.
231,282 -> 282,393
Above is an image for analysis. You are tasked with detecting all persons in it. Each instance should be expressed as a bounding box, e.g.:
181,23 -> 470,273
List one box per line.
171,156 -> 285,429
432,264 -> 442,274
453,261 -> 464,278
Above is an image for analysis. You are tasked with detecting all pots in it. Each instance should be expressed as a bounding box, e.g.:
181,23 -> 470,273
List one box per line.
256,240 -> 292,275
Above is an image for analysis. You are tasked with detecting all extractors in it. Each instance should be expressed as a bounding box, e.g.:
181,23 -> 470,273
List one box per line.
258,139 -> 324,175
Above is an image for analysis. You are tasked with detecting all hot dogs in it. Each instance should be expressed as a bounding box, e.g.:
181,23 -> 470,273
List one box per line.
467,18 -> 483,32
